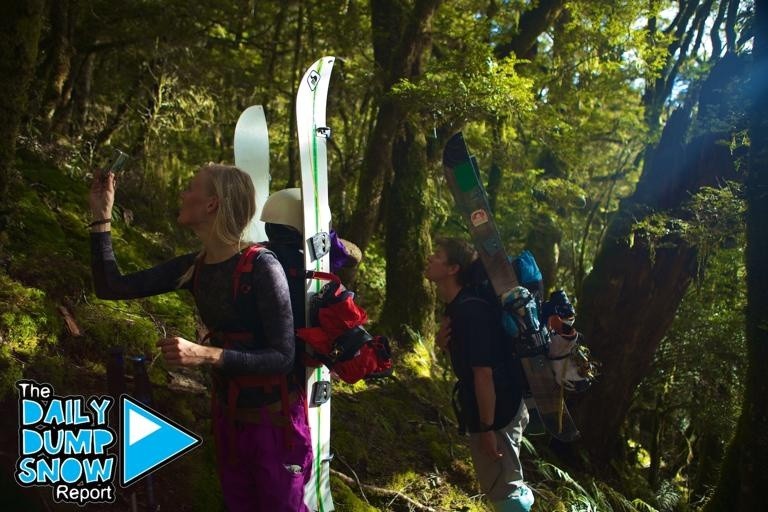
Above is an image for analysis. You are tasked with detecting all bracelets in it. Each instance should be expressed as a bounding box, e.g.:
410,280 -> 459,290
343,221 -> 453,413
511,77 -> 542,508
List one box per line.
88,218 -> 110,227
480,421 -> 495,434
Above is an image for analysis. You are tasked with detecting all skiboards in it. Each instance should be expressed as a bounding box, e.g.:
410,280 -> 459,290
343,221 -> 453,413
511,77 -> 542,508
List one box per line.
441,129 -> 592,466
232,54 -> 338,512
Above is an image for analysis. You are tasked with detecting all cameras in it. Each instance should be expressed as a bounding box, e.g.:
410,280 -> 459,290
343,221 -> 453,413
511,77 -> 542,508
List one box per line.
100,148 -> 130,183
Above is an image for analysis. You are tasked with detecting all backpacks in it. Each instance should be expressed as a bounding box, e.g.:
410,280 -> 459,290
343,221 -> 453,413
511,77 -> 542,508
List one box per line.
193,223 -> 338,383
455,249 -> 547,392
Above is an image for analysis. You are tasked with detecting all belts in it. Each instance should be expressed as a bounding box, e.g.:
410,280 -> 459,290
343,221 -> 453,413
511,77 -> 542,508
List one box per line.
223,388 -> 299,429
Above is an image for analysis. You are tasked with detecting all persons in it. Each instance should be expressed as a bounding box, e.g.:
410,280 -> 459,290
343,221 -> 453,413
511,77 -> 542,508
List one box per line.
421,231 -> 537,512
89,162 -> 315,512
